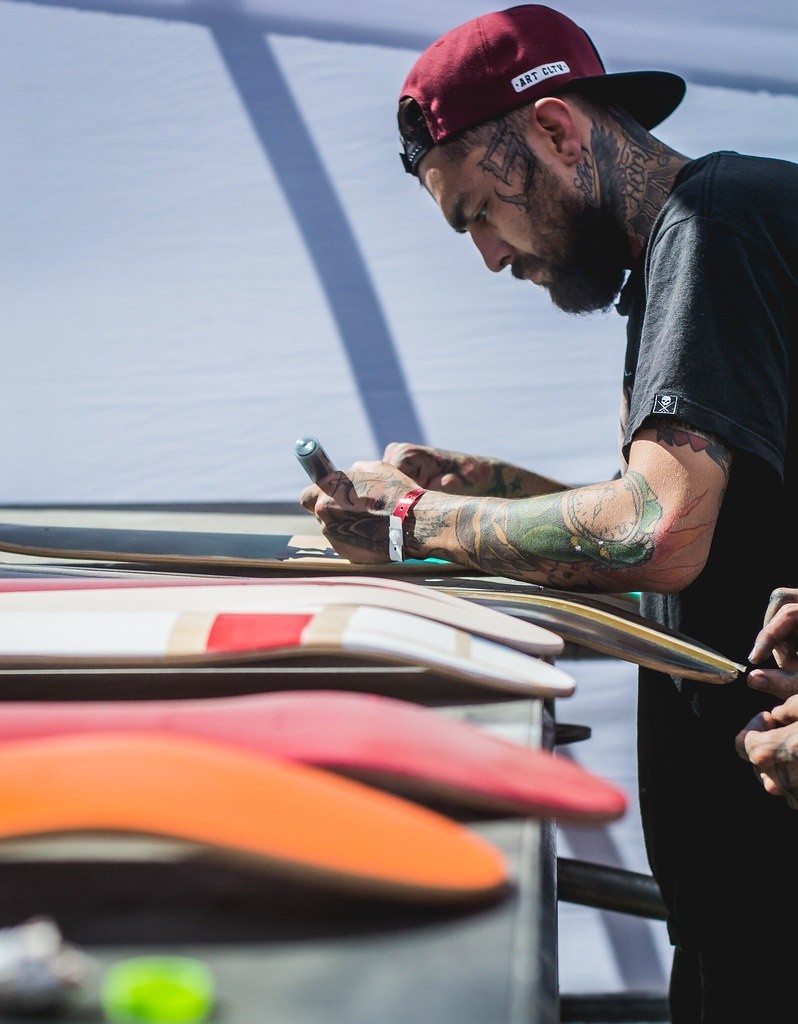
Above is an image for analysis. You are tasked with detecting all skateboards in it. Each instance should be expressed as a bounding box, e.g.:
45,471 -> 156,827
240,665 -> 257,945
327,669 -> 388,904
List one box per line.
0,515 -> 748,908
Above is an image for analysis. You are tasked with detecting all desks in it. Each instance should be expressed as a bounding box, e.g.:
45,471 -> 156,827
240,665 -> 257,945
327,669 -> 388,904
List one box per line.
0,526 -> 558,1022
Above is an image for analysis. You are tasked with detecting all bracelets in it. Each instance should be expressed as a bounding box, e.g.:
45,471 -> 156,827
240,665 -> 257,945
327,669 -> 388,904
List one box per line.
388,489 -> 428,562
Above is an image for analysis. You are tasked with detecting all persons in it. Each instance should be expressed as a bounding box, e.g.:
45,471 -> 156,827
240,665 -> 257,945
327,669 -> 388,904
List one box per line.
299,5 -> 798,1024
734,587 -> 798,810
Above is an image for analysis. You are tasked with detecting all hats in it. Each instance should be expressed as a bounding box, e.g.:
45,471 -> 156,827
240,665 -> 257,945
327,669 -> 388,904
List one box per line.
398,4 -> 687,177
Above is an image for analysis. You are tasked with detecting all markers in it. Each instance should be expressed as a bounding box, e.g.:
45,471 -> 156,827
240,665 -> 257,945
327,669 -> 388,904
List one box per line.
293,434 -> 337,485
